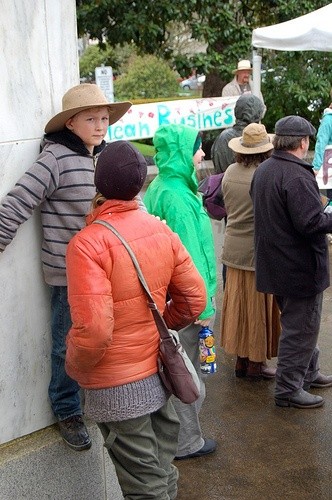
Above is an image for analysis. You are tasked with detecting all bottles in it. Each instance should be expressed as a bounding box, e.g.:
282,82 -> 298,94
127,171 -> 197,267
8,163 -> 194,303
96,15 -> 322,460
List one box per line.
95,64 -> 114,102
198,326 -> 217,374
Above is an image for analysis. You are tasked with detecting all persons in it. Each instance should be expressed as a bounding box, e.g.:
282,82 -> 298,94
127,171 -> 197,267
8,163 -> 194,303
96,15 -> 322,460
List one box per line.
248,116 -> 332,409
222,60 -> 253,96
63,139 -> 205,500
0,83 -> 165,450
313,103 -> 332,241
144,122 -> 220,462
210,91 -> 266,173
219,123 -> 281,380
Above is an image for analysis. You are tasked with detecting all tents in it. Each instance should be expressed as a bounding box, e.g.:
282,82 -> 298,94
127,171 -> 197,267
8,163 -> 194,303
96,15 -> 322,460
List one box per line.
251,4 -> 332,97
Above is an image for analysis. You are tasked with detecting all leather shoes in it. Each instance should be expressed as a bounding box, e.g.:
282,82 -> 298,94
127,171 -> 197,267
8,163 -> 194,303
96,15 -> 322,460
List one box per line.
274,389 -> 325,408
174,439 -> 217,459
59,414 -> 91,452
311,372 -> 332,387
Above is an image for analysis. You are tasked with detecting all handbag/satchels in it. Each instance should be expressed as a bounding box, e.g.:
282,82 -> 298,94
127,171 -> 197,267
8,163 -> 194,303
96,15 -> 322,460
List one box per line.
156,333 -> 200,403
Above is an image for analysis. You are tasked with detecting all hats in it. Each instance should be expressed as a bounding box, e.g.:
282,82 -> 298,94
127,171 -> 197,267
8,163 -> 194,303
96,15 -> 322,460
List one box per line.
232,59 -> 253,74
228,123 -> 275,155
43,84 -> 133,132
275,115 -> 317,135
193,135 -> 203,154
95,140 -> 148,201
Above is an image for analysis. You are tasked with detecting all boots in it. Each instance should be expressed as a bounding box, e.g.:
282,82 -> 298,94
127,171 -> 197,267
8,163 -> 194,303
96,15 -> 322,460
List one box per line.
235,356 -> 249,377
247,357 -> 277,379
